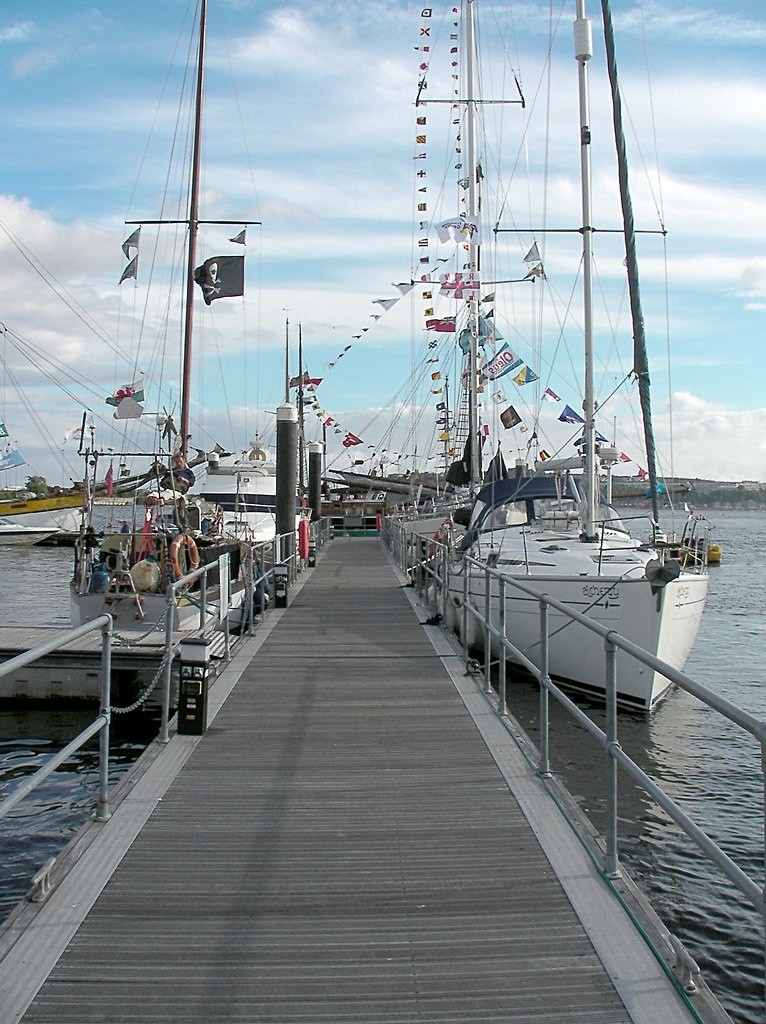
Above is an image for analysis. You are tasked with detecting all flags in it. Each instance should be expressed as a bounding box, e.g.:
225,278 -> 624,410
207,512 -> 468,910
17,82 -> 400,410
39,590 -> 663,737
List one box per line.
106,377 -> 145,408
0,449 -> 26,471
122,227 -> 141,260
0,422 -> 9,438
194,256 -> 244,305
289,6 -> 666,499
228,229 -> 246,244
104,465 -> 113,496
119,254 -> 138,285
64,414 -> 95,441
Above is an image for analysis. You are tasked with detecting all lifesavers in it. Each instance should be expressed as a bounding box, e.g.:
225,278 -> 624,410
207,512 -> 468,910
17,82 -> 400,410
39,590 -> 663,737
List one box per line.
249,449 -> 266,467
376,513 -> 382,532
300,519 -> 309,560
170,533 -> 199,583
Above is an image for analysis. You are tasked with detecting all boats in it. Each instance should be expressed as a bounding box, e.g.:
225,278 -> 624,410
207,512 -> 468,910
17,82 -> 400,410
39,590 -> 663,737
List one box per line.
666,506 -> 721,564
191,428 -> 312,553
0,517 -> 60,544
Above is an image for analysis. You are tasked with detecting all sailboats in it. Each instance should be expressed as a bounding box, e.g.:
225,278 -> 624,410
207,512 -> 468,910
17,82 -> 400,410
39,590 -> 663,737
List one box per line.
0,338 -> 234,543
380,0 -> 710,714
69,0 -> 246,636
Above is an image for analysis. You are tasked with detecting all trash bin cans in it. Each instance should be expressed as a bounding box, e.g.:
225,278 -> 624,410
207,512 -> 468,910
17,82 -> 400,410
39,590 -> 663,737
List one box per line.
309,541 -> 318,567
176,637 -> 210,736
273,564 -> 289,608
330,526 -> 334,539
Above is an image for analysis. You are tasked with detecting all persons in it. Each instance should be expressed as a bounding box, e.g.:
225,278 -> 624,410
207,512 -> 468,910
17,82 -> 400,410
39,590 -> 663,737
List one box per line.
160,455 -> 195,494
121,521 -> 129,533
